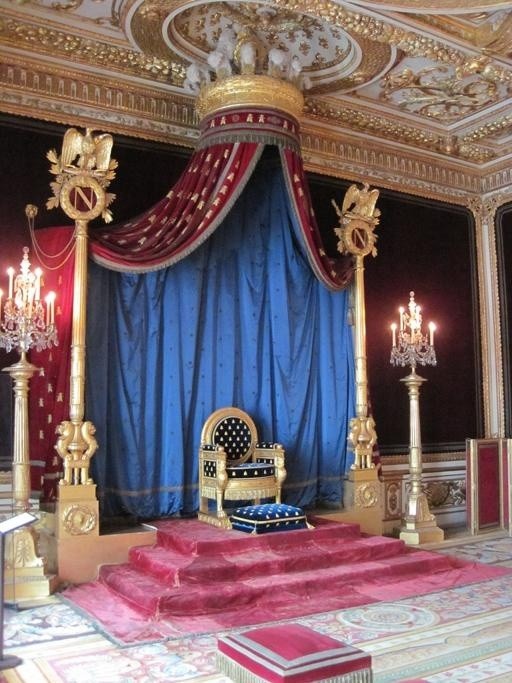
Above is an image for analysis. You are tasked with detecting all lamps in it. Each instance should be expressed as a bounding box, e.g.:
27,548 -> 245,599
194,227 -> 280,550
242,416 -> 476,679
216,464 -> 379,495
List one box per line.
181,7 -> 314,99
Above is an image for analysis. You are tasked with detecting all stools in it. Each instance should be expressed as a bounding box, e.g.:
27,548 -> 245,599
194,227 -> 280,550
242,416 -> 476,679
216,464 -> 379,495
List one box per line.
216,623 -> 372,682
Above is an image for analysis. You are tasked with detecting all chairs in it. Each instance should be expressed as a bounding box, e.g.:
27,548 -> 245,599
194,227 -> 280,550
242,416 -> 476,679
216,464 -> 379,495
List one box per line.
197,407 -> 290,530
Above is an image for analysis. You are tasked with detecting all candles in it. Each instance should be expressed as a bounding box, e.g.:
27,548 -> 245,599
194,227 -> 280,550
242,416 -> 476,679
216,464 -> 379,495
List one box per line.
391,302 -> 436,349
0,258 -> 56,328
